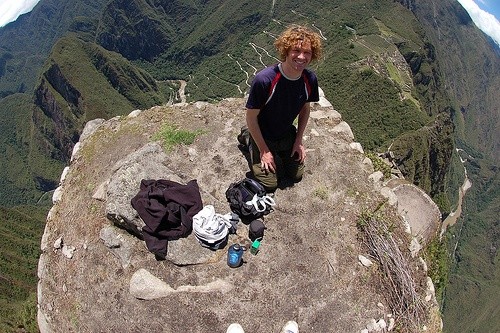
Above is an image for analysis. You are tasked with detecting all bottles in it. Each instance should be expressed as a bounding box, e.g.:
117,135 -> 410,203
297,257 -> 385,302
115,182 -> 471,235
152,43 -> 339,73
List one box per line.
227,244 -> 243,266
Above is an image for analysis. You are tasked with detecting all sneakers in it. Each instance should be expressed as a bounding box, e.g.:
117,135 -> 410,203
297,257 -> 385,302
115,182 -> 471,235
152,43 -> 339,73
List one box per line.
237,125 -> 250,146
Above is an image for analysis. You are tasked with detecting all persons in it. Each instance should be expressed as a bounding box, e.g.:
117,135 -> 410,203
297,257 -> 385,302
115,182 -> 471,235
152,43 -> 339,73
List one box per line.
236,24 -> 322,192
